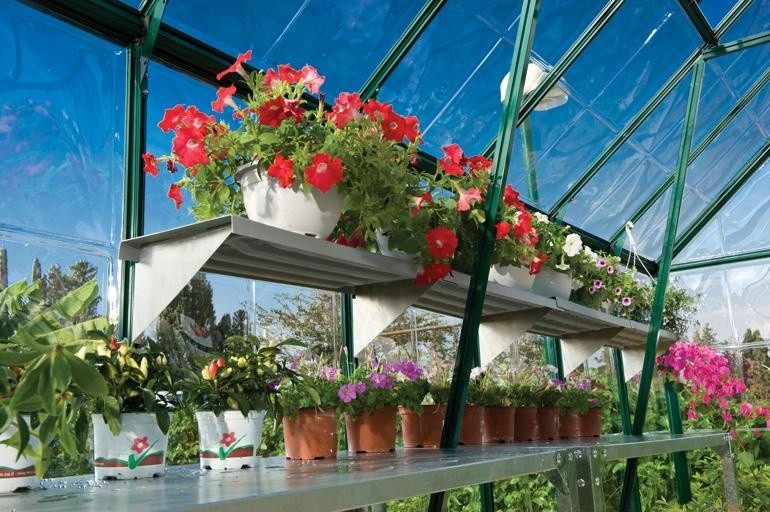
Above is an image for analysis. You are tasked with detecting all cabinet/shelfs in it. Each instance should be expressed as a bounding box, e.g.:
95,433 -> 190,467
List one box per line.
0,210 -> 769,511
